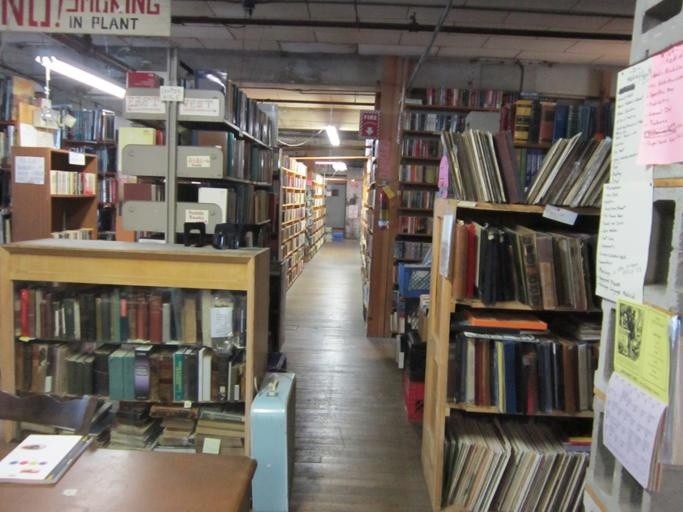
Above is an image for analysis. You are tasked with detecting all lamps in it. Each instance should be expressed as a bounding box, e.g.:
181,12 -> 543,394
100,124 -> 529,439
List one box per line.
35,32 -> 126,98
321,106 -> 350,172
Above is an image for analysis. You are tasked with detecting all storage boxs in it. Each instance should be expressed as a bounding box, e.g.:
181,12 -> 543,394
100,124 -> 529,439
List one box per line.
395,261 -> 429,342
403,367 -> 424,422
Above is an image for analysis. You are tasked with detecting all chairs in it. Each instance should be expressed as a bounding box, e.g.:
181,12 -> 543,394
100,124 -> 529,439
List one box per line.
2,384 -> 121,450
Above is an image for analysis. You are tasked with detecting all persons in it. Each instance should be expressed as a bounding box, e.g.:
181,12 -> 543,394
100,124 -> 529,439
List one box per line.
345,198 -> 358,239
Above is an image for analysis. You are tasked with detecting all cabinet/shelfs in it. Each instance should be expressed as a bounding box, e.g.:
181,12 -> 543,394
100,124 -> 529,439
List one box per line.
0,234 -> 273,459
0,48 -> 328,295
421,192 -> 605,511
360,55 -> 619,340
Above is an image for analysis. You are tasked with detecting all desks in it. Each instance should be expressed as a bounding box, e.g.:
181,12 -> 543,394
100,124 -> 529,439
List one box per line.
0,442 -> 260,512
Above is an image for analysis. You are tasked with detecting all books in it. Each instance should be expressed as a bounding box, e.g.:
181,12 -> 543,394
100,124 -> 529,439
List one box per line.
12,285 -> 247,448
0,70 -> 272,248
358,87 -> 682,510
280,157 -> 325,289
0,433 -> 93,485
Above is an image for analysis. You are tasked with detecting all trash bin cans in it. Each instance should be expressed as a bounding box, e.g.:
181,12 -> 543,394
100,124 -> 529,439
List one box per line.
405,349 -> 426,421
